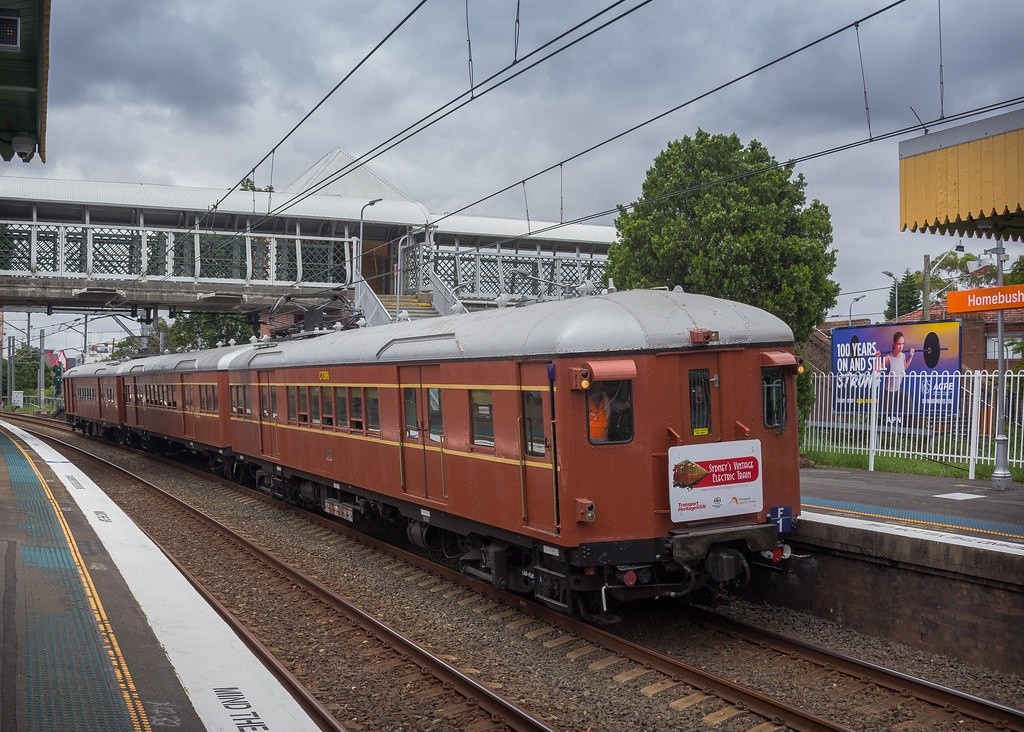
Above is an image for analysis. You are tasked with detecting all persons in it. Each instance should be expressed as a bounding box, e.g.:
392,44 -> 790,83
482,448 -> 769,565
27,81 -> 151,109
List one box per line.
693,385 -> 711,429
588,381 -> 631,442
872,331 -> 914,413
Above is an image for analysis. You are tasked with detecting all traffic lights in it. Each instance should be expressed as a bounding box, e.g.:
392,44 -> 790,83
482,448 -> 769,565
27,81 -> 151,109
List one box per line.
55,367 -> 61,386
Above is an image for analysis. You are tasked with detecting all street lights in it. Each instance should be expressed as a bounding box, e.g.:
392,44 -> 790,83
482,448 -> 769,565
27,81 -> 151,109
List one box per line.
882,271 -> 899,322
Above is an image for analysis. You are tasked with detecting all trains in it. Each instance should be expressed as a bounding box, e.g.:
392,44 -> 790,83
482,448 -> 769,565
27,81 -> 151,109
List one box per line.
64,293 -> 821,633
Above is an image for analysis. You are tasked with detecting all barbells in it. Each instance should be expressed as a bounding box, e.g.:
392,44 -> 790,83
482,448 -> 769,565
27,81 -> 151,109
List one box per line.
848,332 -> 948,373
